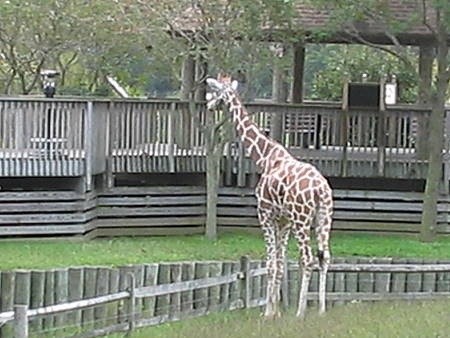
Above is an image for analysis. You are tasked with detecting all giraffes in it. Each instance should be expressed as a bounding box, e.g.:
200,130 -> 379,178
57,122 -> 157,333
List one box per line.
205,72 -> 333,321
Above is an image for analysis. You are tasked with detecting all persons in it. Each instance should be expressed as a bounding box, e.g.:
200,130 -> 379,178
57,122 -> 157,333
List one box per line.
384,74 -> 397,105
43,74 -> 55,97
